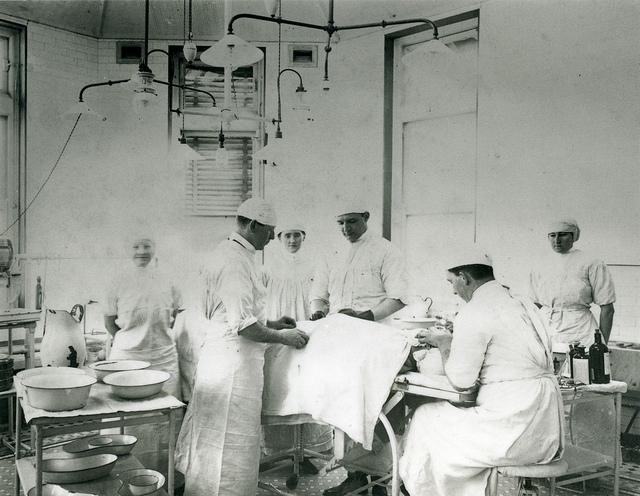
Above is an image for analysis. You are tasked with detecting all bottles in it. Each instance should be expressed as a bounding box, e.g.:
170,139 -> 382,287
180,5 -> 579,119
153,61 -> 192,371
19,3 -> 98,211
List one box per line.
566,327 -> 612,385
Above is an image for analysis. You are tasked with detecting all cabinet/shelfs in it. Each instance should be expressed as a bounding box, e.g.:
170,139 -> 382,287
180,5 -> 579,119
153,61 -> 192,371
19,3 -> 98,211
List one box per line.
12,372 -> 187,495
0,319 -> 38,460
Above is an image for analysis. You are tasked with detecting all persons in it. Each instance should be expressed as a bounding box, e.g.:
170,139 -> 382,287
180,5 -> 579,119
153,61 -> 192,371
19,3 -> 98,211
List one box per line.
308,198 -> 410,496
174,196 -> 309,496
101,230 -> 189,494
398,247 -> 565,496
260,210 -> 335,475
527,214 -> 616,371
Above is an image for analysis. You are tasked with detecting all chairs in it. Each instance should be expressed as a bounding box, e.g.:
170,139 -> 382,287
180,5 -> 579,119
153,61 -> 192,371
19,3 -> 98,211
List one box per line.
492,378 -> 624,495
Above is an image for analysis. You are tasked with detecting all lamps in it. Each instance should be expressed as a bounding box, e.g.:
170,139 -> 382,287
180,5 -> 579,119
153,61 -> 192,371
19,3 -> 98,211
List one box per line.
202,0 -> 462,116
56,0 -> 237,128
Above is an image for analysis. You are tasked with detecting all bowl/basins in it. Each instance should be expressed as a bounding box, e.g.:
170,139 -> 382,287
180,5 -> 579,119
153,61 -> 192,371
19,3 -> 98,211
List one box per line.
401,317 -> 437,329
129,474 -> 159,494
88,436 -> 113,450
16,356 -> 171,411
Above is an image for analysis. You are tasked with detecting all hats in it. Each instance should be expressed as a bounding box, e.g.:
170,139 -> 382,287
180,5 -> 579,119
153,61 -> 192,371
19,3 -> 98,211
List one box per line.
333,197 -> 368,216
236,198 -> 277,227
276,224 -> 305,235
446,246 -> 492,270
546,220 -> 580,242
